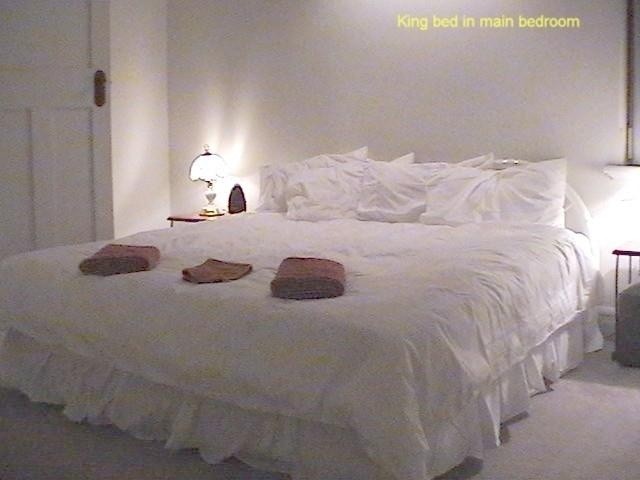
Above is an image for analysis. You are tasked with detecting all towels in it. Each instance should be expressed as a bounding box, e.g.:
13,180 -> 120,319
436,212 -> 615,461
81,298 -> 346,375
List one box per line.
78,243 -> 346,301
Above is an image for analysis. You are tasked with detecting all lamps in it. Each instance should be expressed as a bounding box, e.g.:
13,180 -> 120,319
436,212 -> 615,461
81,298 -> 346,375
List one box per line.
189,146 -> 227,218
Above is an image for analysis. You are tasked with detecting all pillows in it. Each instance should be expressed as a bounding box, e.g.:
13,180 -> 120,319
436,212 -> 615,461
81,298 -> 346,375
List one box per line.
259,146 -> 592,233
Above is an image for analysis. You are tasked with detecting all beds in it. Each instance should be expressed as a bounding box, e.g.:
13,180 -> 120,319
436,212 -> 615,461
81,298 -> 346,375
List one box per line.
0,146 -> 595,480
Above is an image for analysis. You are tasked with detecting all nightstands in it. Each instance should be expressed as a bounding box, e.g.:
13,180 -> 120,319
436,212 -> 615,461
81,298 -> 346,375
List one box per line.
168,212 -> 213,227
